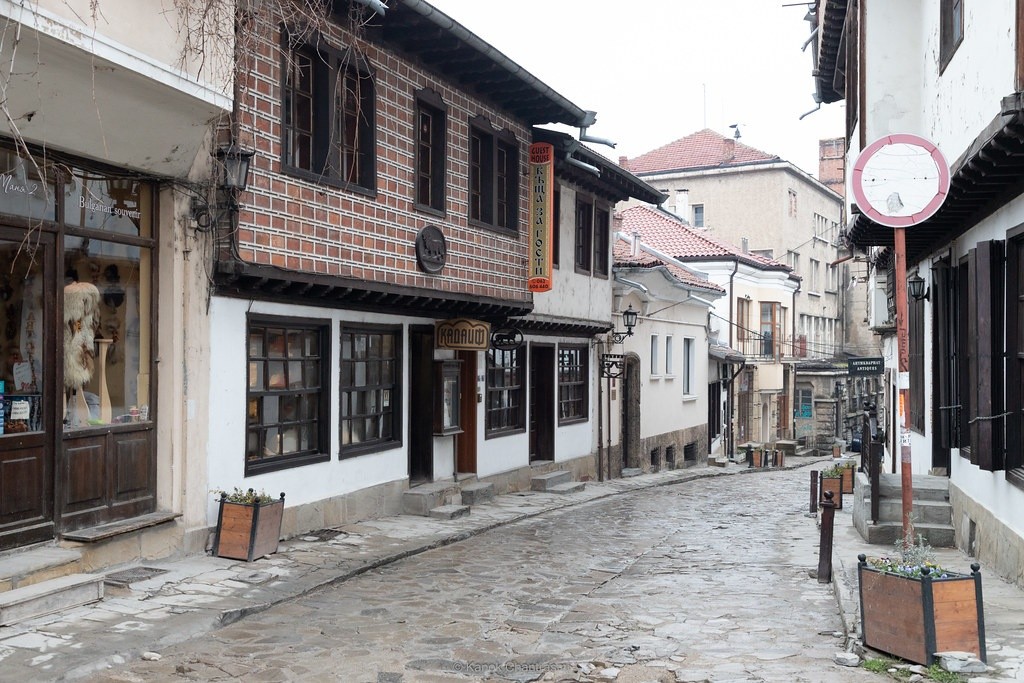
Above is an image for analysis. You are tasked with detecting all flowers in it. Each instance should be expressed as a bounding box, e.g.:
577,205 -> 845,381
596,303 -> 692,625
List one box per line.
863,513 -> 950,578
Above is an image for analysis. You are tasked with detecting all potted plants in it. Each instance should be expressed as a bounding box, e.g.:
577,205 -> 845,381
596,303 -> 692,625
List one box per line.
832,443 -> 843,458
835,462 -> 857,494
819,466 -> 844,509
208,486 -> 285,564
737,447 -> 786,468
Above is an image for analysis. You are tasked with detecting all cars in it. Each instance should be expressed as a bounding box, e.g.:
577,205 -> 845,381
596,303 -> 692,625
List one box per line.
852,429 -> 864,452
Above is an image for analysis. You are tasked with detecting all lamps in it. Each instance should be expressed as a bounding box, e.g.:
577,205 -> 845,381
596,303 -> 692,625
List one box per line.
930,255 -> 958,291
908,270 -> 930,303
611,301 -> 640,344
191,138 -> 257,234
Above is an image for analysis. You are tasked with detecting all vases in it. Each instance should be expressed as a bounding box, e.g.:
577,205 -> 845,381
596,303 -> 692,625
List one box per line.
857,554 -> 988,666
